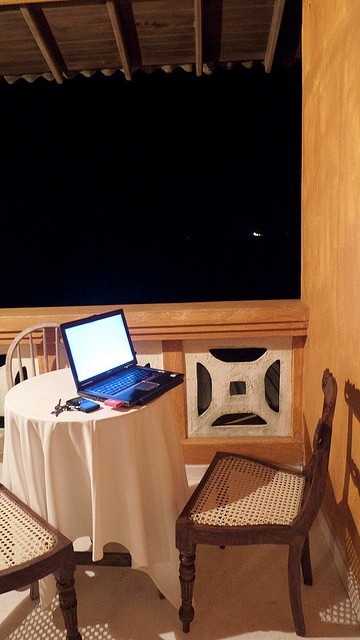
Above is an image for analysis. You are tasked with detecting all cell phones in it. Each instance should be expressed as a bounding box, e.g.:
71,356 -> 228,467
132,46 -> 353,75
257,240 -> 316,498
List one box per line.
66,397 -> 100,413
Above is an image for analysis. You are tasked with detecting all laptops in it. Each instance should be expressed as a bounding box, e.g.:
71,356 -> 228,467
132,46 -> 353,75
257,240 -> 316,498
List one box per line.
60,309 -> 185,407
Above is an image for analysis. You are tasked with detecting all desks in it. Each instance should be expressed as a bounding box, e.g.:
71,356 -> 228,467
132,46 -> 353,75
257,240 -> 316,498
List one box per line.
4,367 -> 192,600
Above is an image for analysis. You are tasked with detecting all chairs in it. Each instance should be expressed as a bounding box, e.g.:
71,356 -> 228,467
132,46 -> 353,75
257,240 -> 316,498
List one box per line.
6,323 -> 71,391
175,368 -> 338,637
0,483 -> 82,640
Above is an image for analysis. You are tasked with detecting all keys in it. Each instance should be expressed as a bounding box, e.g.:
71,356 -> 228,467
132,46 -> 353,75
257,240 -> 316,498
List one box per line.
50,398 -> 75,418
